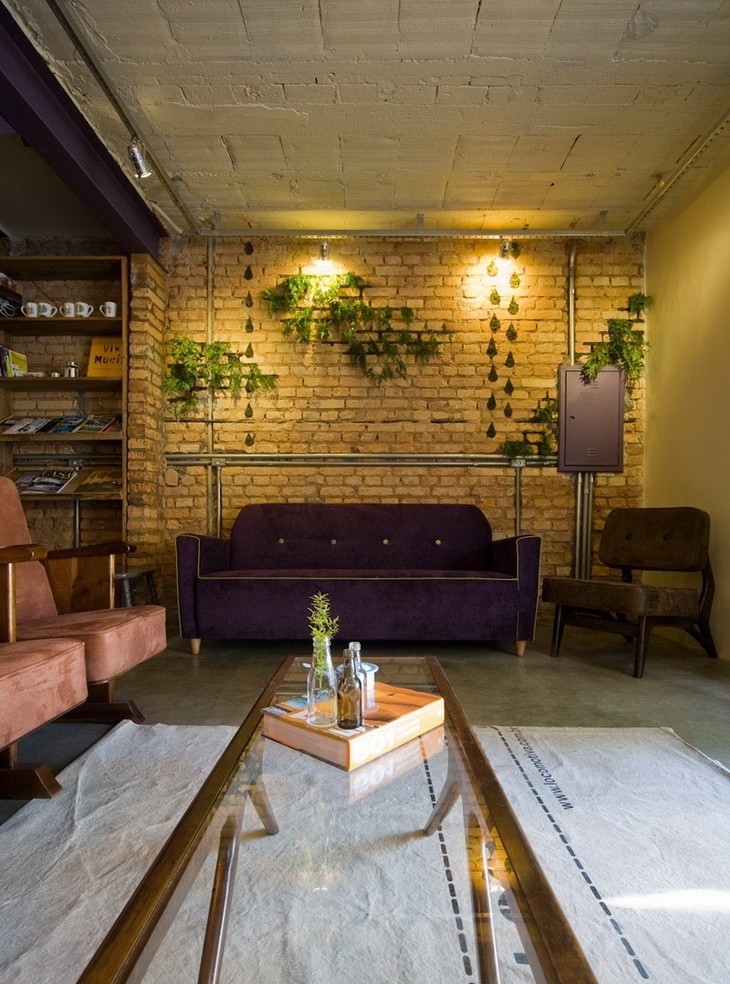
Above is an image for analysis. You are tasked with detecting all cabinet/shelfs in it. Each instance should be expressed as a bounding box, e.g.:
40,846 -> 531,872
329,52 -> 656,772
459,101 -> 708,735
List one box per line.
0,254 -> 128,574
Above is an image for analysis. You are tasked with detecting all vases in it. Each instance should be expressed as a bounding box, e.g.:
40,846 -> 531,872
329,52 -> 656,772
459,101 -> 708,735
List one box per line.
304,635 -> 340,728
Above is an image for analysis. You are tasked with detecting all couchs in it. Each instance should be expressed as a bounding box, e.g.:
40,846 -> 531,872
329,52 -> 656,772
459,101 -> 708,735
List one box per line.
175,501 -> 542,657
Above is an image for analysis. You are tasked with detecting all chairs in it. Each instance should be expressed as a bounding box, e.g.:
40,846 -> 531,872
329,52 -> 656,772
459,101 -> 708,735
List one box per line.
541,507 -> 717,679
0,636 -> 89,802
0,476 -> 170,726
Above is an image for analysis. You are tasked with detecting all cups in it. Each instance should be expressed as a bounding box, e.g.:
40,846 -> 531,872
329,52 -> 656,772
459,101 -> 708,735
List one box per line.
37,302 -> 58,318
74,302 -> 94,318
59,302 -> 74,317
99,301 -> 117,317
21,302 -> 37,318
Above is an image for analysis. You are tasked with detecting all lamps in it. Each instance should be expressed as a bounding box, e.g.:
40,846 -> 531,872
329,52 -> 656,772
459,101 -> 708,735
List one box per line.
320,242 -> 330,262
127,138 -> 153,181
500,240 -> 511,262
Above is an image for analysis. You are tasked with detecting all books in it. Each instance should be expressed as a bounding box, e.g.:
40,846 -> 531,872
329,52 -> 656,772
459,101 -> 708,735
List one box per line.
261,726 -> 445,806
258,680 -> 445,772
0,413 -> 122,434
0,344 -> 28,378
13,468 -> 122,494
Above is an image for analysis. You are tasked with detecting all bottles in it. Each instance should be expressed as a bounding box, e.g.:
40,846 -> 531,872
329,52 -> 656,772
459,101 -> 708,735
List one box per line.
337,649 -> 363,729
63,361 -> 79,378
339,641 -> 367,721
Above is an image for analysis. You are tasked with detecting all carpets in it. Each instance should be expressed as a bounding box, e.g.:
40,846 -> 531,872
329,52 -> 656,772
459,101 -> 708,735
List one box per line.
0,719 -> 730,984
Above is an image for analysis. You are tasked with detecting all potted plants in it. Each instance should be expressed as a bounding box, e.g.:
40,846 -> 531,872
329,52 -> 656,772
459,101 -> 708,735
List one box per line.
75,651 -> 598,984
